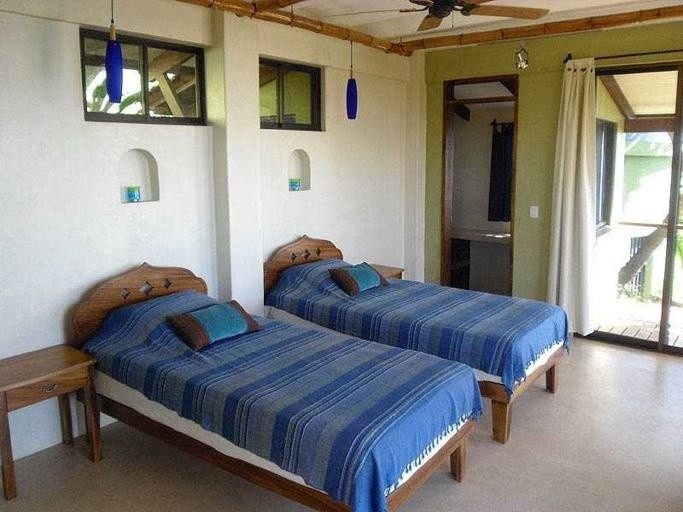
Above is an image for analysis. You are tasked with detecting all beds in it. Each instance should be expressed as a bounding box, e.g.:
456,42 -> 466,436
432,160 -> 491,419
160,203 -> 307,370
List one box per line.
263,235 -> 570,444
71,262 -> 483,512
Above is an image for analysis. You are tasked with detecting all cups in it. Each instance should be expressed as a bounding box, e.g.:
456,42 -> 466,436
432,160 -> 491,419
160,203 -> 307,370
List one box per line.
127,185 -> 140,203
290,178 -> 300,191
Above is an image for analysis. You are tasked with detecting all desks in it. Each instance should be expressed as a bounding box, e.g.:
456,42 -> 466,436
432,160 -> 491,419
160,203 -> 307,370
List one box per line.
452,229 -> 511,296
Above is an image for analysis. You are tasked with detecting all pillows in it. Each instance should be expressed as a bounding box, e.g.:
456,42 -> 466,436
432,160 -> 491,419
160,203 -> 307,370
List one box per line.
328,263 -> 390,296
167,300 -> 261,352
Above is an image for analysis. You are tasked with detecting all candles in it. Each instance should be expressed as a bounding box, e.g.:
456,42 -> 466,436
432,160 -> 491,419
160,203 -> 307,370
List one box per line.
291,177 -> 300,190
129,186 -> 140,202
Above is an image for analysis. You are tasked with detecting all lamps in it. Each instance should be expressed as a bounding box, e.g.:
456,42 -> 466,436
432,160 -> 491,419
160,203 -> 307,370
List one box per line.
514,47 -> 529,70
346,38 -> 357,121
104,1 -> 122,102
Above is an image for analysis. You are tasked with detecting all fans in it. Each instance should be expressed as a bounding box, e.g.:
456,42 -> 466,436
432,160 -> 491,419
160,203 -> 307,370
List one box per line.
324,0 -> 549,31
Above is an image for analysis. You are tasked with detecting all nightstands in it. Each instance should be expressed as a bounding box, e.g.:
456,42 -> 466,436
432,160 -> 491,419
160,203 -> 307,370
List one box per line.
369,263 -> 404,279
0,344 -> 105,500
